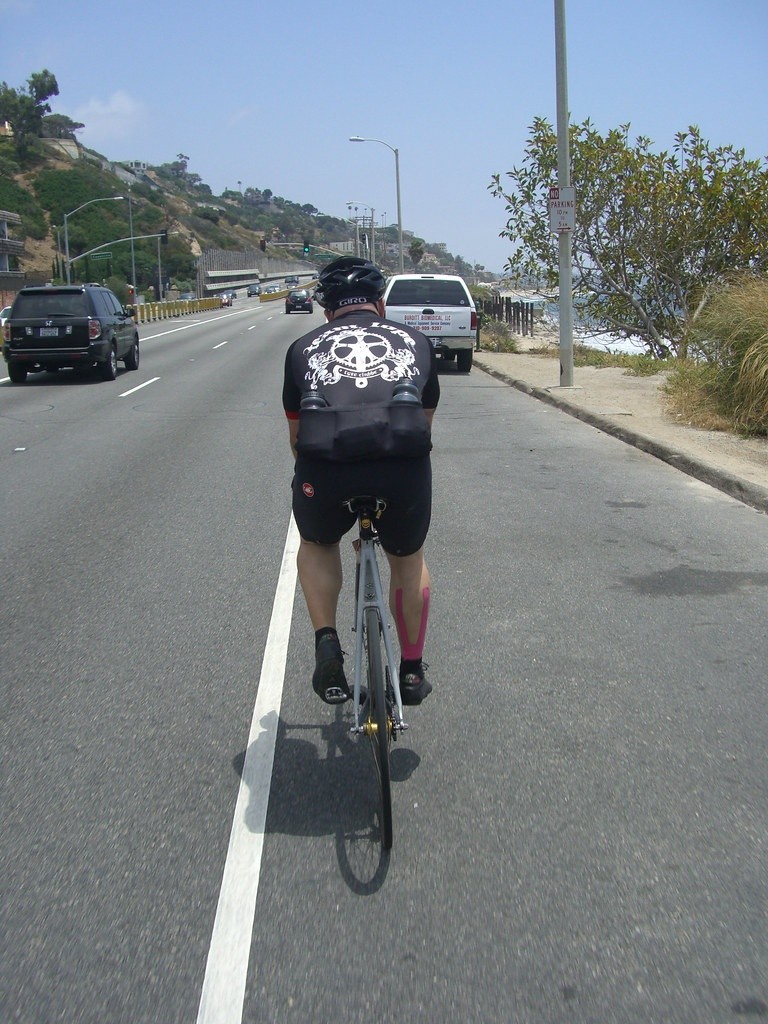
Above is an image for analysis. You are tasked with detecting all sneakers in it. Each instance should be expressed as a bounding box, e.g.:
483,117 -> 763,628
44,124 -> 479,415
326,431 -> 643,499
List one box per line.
400,659 -> 432,704
313,633 -> 352,703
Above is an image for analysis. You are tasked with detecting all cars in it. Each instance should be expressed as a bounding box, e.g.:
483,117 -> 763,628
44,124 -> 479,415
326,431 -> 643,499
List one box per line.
224,289 -> 237,299
264,275 -> 298,295
248,284 -> 262,296
312,273 -> 321,279
214,293 -> 232,307
176,292 -> 195,301
284,288 -> 316,314
0,305 -> 12,326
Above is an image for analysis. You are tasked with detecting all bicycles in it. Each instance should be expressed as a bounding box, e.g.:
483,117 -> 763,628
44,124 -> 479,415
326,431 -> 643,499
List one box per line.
322,495 -> 418,854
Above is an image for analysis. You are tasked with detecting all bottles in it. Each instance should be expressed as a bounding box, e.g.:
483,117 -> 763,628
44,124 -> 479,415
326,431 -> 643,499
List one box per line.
300,388 -> 325,410
391,377 -> 420,404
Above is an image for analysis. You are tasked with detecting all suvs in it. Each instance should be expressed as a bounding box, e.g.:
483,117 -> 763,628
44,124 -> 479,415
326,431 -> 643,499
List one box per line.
2,283 -> 140,384
380,274 -> 478,373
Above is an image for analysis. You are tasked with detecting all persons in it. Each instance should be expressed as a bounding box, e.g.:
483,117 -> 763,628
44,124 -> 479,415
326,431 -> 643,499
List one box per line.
282,256 -> 440,705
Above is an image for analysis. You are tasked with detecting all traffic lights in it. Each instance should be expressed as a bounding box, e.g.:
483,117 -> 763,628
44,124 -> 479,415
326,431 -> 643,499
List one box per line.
304,240 -> 310,252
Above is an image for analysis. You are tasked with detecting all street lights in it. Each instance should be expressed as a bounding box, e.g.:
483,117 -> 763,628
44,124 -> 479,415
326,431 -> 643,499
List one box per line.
346,200 -> 378,266
63,196 -> 124,287
348,135 -> 403,273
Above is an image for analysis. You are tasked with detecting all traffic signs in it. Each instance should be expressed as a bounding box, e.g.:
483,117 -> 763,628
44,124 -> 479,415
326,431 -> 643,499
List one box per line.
549,186 -> 577,232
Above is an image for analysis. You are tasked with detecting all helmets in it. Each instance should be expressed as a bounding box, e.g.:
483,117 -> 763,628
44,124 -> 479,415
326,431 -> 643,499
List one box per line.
311,256 -> 388,308
